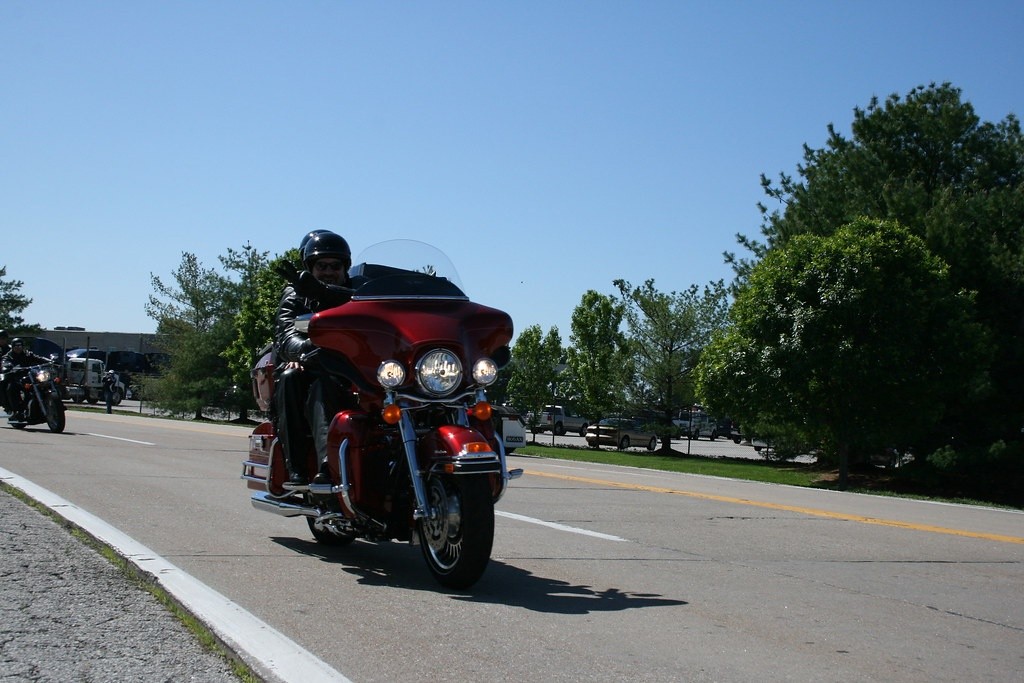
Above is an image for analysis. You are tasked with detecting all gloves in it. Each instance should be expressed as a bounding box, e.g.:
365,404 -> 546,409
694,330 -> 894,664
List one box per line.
2,364 -> 13,374
44,360 -> 53,367
285,335 -> 329,361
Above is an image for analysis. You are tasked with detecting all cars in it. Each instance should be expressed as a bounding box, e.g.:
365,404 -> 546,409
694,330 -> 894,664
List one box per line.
718,419 -> 776,451
127,386 -> 142,400
584,416 -> 657,451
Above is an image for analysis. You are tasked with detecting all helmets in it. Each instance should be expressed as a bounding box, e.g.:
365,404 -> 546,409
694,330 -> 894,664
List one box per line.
11,338 -> 24,346
303,232 -> 351,272
299,230 -> 332,254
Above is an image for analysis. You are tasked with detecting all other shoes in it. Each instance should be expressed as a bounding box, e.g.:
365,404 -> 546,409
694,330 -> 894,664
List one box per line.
286,464 -> 311,481
9,410 -> 24,422
314,464 -> 332,485
63,405 -> 67,411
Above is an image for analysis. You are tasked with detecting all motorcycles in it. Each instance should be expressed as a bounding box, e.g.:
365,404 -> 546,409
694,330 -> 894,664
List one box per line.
0,354 -> 68,432
240,239 -> 516,587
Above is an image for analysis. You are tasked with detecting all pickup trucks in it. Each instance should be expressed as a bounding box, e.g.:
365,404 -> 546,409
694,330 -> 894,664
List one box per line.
525,405 -> 589,436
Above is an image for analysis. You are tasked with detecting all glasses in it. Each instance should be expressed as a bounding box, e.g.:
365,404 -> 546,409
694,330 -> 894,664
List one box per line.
312,262 -> 344,272
12,345 -> 23,349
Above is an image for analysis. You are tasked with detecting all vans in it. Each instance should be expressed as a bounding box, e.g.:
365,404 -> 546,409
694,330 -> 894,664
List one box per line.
672,406 -> 717,441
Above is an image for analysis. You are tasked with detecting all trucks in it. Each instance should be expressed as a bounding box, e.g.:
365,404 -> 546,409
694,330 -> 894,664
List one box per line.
488,402 -> 527,454
0,329 -> 126,407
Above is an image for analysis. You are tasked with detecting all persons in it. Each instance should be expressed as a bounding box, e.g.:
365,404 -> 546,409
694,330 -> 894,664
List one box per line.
102,370 -> 117,415
271,230 -> 353,486
0,329 -> 54,422
